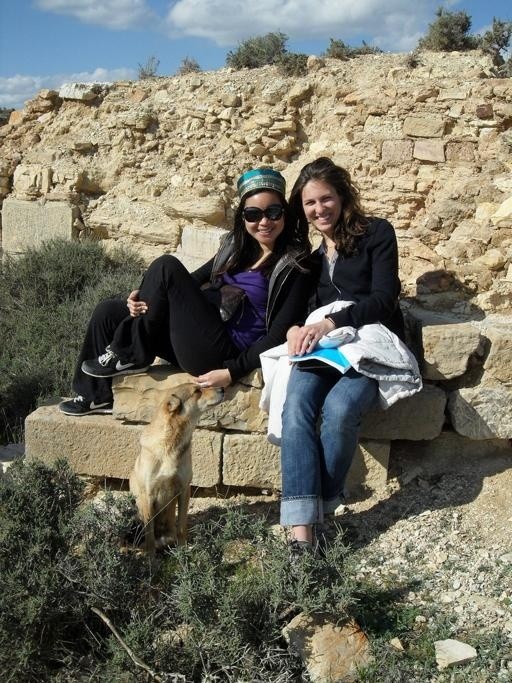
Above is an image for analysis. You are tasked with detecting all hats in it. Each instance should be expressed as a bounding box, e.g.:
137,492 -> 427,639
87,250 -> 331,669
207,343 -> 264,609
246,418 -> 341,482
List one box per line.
237,169 -> 285,198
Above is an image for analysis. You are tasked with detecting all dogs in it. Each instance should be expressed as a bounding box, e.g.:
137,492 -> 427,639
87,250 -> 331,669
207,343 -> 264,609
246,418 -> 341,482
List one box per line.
129,380 -> 227,558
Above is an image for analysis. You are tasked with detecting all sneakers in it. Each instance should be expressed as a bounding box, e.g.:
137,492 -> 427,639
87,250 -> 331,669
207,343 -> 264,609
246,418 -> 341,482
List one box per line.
288,539 -> 314,580
81,345 -> 149,377
59,396 -> 114,415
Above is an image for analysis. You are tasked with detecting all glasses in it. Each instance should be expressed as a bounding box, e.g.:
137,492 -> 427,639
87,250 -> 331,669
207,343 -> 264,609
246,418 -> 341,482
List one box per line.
243,204 -> 285,222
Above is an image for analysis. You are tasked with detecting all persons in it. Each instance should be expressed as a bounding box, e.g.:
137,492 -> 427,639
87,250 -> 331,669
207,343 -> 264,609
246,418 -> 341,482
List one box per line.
57,166 -> 314,418
279,156 -> 407,559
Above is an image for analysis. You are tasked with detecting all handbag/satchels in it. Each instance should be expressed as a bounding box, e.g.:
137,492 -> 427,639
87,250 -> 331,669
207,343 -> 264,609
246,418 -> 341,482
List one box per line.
199,282 -> 246,322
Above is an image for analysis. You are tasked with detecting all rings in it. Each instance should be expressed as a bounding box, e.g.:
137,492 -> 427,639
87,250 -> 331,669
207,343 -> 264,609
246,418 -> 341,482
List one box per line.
309,332 -> 313,338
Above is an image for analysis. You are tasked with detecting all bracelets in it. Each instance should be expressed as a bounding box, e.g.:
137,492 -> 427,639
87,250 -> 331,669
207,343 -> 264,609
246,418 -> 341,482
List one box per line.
327,317 -> 336,328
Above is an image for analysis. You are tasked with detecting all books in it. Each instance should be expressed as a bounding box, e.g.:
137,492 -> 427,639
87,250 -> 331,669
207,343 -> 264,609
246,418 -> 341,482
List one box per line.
290,348 -> 352,375
